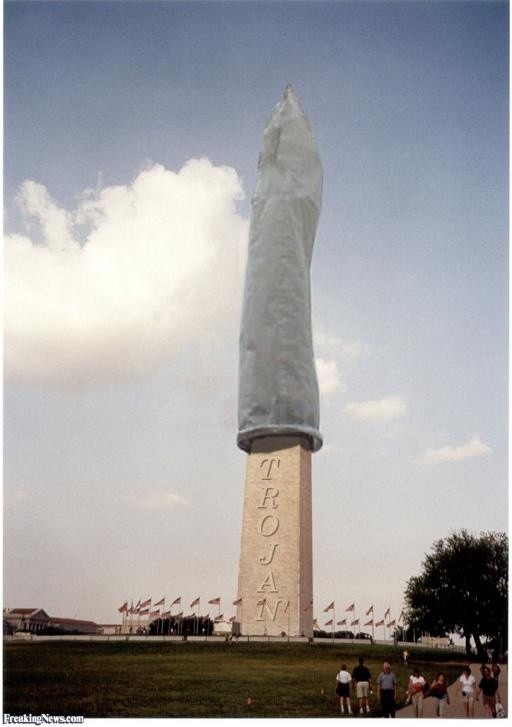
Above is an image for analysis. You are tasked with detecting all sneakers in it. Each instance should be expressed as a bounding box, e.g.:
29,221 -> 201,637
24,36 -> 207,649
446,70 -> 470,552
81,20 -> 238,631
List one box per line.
360,706 -> 370,714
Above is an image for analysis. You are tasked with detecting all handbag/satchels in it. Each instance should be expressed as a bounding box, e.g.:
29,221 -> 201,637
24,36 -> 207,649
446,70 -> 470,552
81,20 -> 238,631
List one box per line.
496,703 -> 505,718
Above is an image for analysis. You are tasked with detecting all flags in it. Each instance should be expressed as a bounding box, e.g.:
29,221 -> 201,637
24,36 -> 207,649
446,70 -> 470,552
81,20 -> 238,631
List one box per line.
118,596 -> 396,639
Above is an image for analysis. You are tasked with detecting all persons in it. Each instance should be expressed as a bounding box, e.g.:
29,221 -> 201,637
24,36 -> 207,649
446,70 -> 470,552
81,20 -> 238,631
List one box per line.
429,672 -> 450,718
376,661 -> 397,718
455,666 -> 476,717
336,664 -> 356,714
405,669 -> 425,718
225,631 -> 240,645
477,660 -> 501,718
351,657 -> 373,714
403,648 -> 408,664
